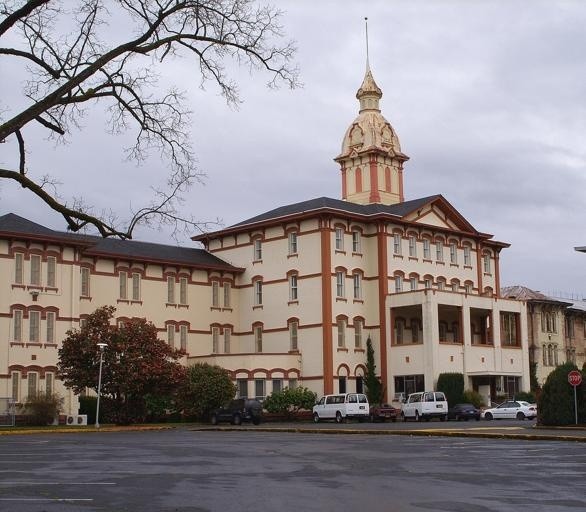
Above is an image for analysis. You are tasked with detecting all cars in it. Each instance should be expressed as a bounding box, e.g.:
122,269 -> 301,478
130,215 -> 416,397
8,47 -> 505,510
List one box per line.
481,400 -> 537,421
448,403 -> 480,422
369,404 -> 396,423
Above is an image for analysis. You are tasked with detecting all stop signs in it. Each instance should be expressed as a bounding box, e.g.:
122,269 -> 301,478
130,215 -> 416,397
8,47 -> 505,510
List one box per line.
567,371 -> 582,425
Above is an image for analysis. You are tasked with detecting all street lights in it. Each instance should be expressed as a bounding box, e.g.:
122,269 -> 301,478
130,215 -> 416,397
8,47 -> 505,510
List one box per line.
93,343 -> 108,428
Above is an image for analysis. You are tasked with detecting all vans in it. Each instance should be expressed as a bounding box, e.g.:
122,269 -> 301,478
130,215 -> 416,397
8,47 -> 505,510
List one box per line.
400,392 -> 448,422
313,393 -> 369,423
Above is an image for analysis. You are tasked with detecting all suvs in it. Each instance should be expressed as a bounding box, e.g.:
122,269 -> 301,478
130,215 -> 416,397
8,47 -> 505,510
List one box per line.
207,397 -> 262,426
